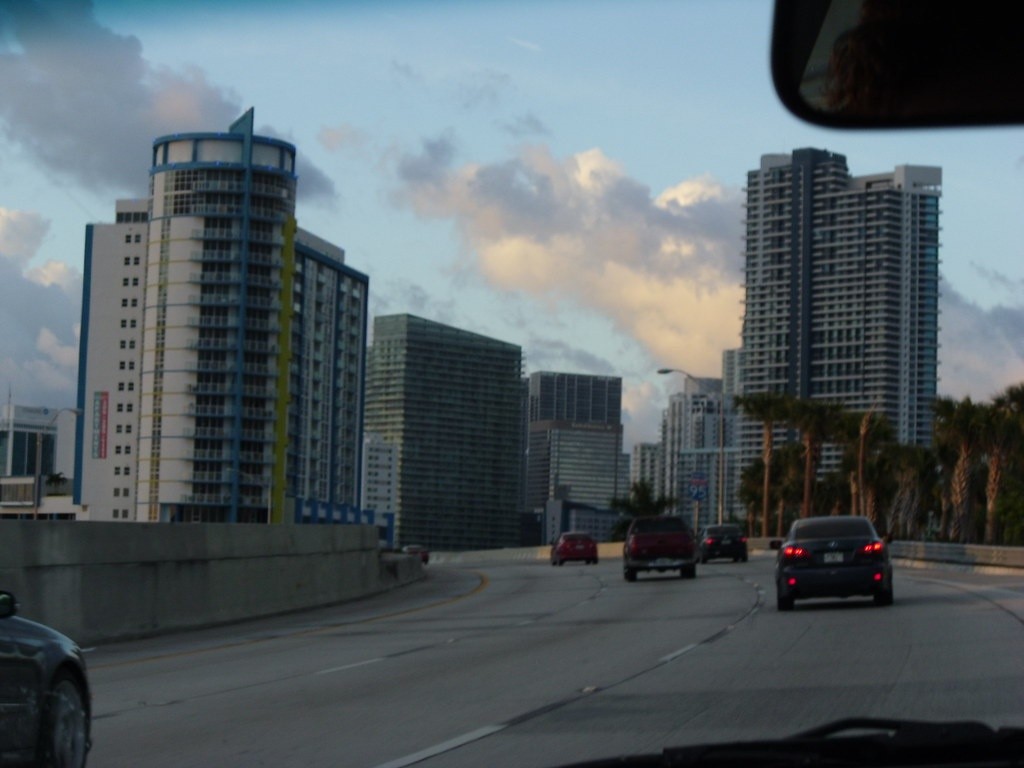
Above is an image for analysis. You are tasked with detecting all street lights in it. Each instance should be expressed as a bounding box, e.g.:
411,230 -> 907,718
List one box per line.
657,368 -> 724,525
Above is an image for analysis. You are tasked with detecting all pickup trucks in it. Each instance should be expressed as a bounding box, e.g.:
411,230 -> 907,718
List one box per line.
622,514 -> 700,581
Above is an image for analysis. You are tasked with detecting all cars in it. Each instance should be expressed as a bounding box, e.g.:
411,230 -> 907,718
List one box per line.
549,531 -> 599,567
769,516 -> 896,609
698,524 -> 749,564
0,591 -> 94,768
